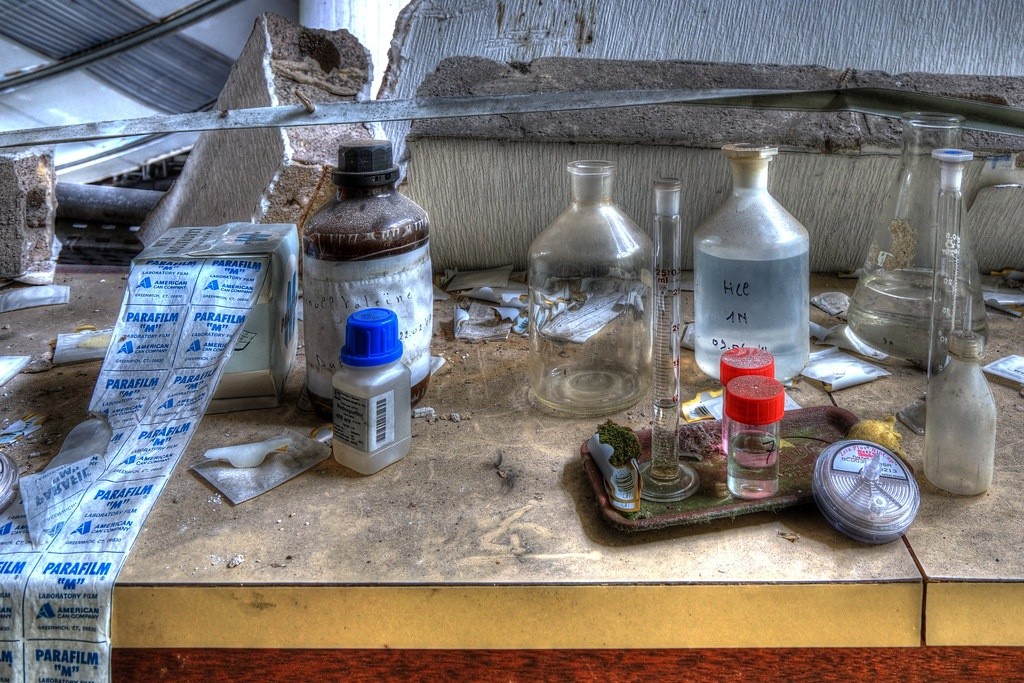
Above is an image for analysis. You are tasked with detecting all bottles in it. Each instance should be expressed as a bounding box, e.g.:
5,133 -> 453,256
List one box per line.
527,159 -> 658,421
719,345 -> 787,500
843,107 -> 991,366
689,142 -> 813,385
330,305 -> 413,477
301,136 -> 435,424
921,293 -> 1000,500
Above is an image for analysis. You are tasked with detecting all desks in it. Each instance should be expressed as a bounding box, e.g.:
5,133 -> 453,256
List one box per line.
0,265 -> 1024,682
